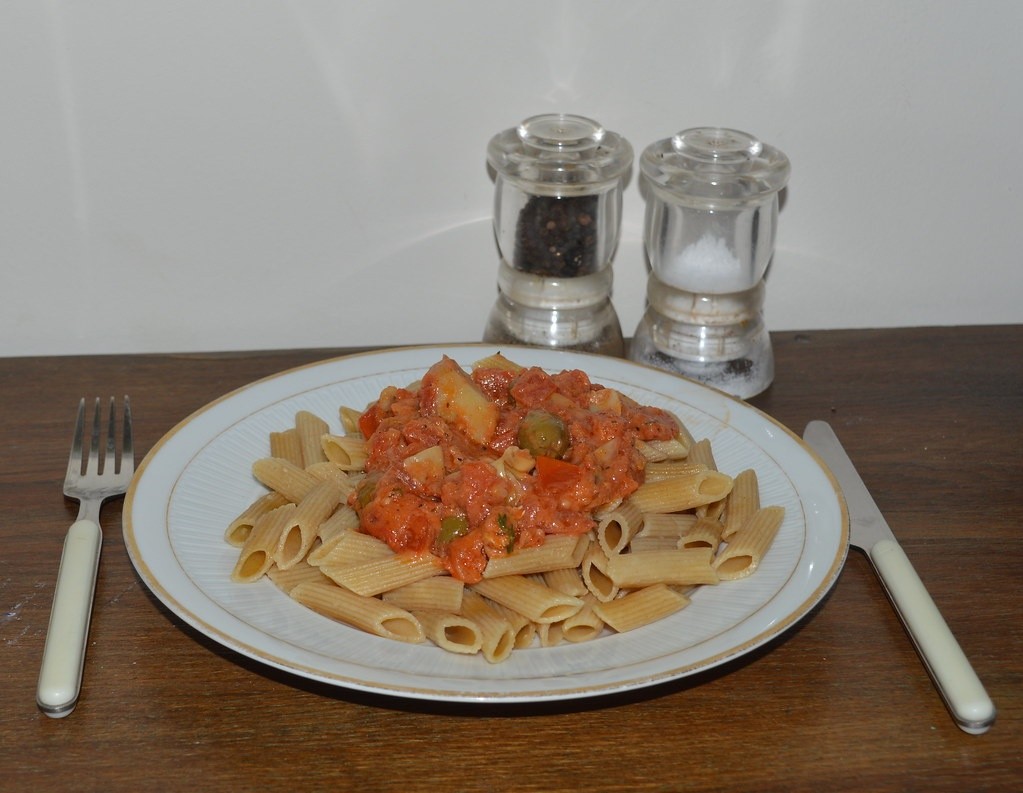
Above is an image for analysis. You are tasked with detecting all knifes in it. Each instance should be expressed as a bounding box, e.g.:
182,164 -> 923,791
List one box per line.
801,420 -> 995,735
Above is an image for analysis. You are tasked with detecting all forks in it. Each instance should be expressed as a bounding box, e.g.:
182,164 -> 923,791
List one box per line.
34,397 -> 134,717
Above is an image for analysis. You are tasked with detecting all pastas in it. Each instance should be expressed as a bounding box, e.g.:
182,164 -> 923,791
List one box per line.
224,350 -> 785,665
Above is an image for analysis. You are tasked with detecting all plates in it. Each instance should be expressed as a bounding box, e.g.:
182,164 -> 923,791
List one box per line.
122,345 -> 851,702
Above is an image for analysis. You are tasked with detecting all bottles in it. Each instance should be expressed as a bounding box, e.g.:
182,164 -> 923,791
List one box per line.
629,124 -> 792,406
482,114 -> 627,361
